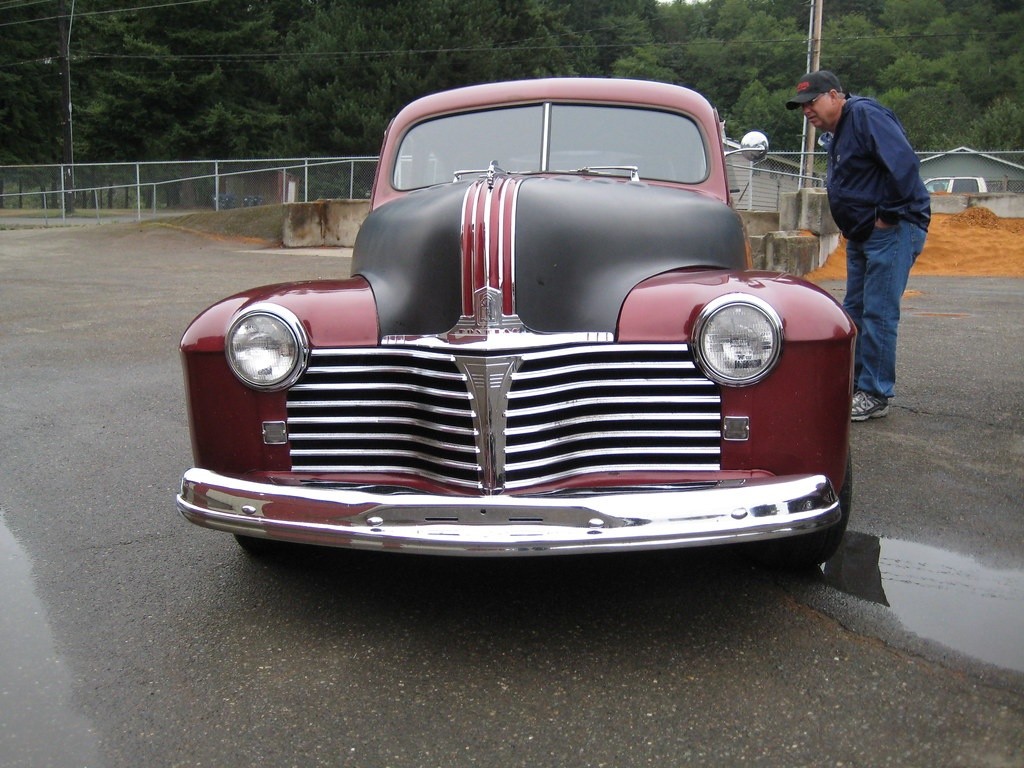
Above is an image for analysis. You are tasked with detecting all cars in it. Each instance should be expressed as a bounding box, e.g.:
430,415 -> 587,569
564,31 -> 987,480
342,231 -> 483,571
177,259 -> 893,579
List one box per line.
179,78 -> 857,568
923,176 -> 988,192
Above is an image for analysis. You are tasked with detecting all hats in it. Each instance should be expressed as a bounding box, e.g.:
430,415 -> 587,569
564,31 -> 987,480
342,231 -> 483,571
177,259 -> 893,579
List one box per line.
785,72 -> 842,110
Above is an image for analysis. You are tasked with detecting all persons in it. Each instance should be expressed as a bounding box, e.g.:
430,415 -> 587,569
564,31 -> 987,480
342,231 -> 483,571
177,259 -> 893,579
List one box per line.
785,71 -> 932,421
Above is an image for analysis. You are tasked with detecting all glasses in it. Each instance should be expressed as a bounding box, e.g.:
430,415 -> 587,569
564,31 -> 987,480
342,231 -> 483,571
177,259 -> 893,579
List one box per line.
800,93 -> 824,108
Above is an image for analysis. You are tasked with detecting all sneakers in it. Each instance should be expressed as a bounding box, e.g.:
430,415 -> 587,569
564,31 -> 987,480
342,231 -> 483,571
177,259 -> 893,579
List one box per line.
849,389 -> 889,420
